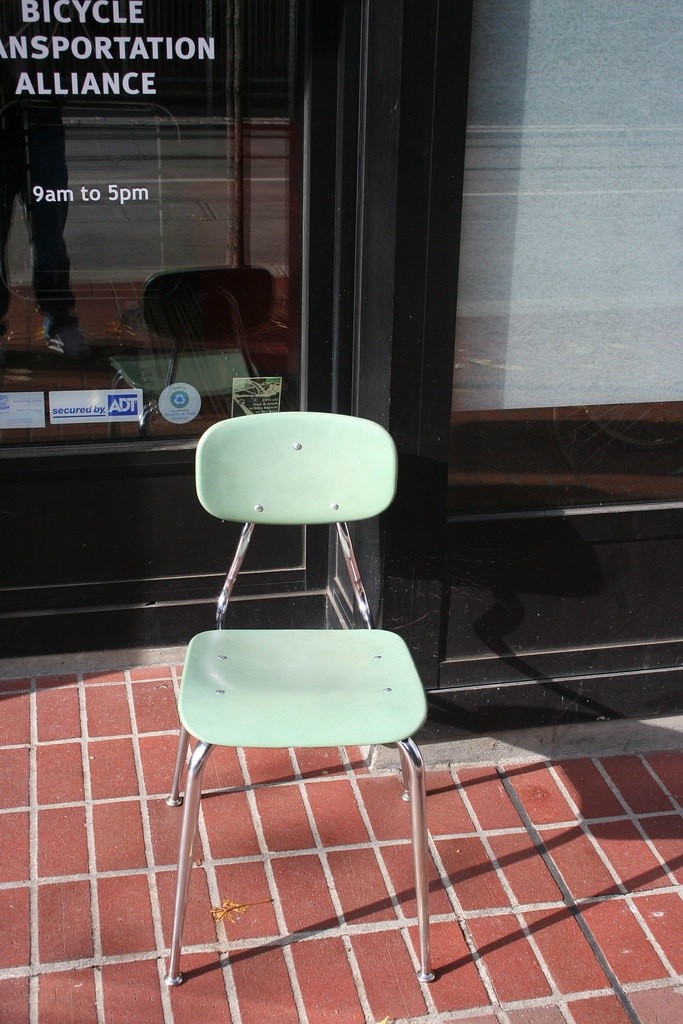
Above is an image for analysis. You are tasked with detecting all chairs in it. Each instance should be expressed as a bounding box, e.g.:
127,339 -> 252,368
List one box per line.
161,408 -> 442,990
110,264 -> 276,435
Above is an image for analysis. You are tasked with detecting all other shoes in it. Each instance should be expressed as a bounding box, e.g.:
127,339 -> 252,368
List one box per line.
44,326 -> 92,360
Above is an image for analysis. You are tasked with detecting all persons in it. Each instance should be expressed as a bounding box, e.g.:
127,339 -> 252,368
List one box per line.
2,0 -> 93,371
